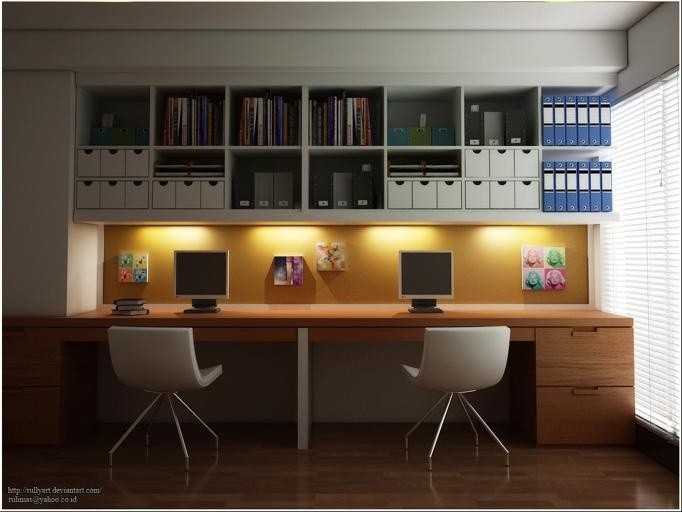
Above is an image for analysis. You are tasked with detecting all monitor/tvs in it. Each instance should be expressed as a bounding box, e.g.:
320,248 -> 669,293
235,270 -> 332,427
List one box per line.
398,250 -> 455,313
174,249 -> 229,313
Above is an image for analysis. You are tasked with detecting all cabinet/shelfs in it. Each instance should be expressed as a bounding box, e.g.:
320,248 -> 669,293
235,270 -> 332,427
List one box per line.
72,70 -> 620,226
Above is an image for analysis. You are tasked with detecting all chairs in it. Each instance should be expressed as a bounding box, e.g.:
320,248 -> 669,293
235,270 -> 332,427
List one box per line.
105,326 -> 224,472
400,324 -> 511,472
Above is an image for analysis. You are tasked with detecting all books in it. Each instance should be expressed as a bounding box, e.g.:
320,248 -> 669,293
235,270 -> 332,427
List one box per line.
159,92 -> 382,145
112,296 -> 150,316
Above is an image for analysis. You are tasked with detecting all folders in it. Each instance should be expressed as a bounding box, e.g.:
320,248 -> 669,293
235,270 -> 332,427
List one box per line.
542,160 -> 612,212
541,95 -> 611,146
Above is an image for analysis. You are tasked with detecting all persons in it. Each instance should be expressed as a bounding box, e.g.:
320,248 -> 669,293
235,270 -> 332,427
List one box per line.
524,271 -> 543,290
522,249 -> 544,268
545,248 -> 565,268
545,269 -> 565,289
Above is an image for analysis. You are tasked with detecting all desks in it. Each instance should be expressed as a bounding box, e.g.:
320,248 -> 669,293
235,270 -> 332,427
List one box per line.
2,303 -> 634,452
